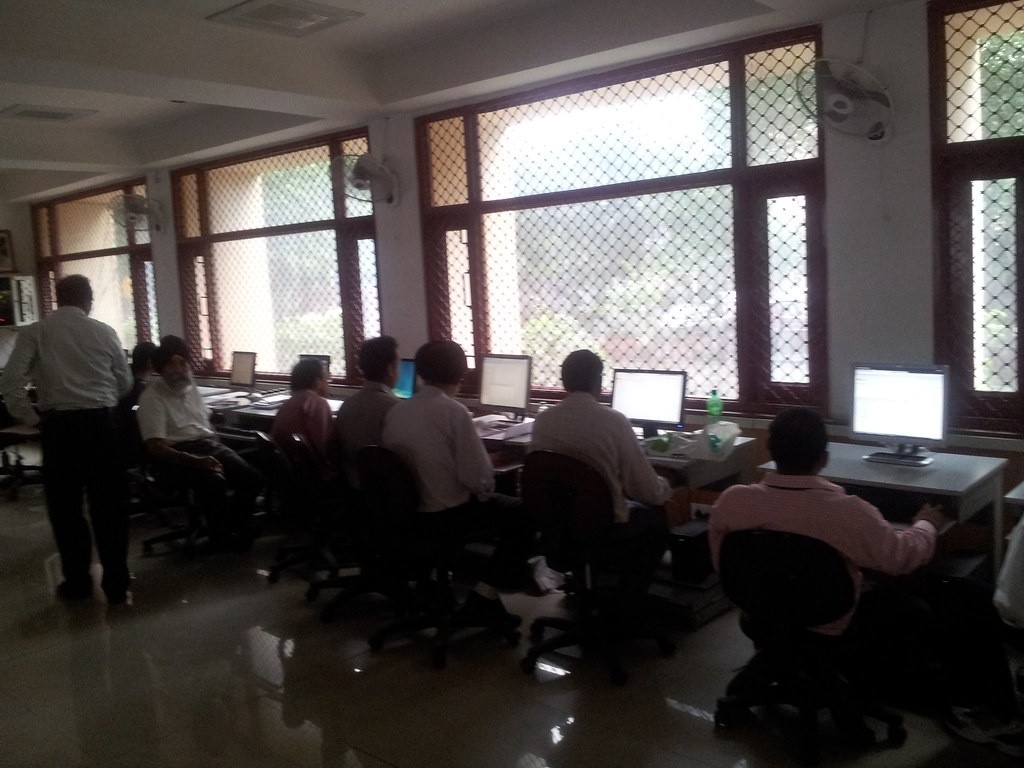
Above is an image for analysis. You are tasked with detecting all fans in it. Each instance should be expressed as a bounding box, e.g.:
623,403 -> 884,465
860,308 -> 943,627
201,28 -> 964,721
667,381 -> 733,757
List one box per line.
326,149 -> 403,204
106,193 -> 169,233
795,59 -> 896,139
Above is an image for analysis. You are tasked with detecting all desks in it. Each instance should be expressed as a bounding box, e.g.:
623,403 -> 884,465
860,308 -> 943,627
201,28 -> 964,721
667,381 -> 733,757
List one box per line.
191,384 -> 346,420
506,431 -> 758,526
467,412 -> 535,440
759,441 -> 1008,573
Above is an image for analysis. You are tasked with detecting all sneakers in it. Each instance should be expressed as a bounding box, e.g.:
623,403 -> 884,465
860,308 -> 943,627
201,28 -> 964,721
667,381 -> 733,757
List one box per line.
943,705 -> 1024,757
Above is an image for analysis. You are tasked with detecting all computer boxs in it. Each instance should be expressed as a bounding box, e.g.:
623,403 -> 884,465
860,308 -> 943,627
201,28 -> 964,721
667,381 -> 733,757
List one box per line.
928,546 -> 996,665
668,519 -> 714,585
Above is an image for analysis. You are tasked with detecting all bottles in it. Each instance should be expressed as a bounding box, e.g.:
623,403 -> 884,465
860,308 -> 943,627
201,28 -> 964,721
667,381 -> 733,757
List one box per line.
707,390 -> 723,422
537,401 -> 549,414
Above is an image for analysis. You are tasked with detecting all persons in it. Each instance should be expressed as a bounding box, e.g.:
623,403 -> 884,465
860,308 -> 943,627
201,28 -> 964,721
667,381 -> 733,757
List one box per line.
337,338 -> 398,487
117,338 -> 265,552
532,350 -> 673,626
710,408 -> 944,700
382,341 -> 527,626
268,359 -> 332,464
0,276 -> 130,591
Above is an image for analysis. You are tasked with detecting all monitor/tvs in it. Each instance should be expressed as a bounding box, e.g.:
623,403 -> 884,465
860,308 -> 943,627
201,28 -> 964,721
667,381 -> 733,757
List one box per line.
849,361 -> 949,467
300,355 -> 330,376
392,358 -> 416,399
230,351 -> 257,397
610,369 -> 688,438
477,353 -> 531,426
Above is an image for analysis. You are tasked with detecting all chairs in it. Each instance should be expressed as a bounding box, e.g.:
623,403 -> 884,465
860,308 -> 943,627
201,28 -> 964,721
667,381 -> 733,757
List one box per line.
140,438 -> 521,668
714,526 -> 910,755
514,450 -> 684,680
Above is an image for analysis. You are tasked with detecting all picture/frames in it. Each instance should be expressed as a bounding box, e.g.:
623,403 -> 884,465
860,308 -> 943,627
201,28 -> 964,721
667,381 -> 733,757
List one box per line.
0,230 -> 17,272
